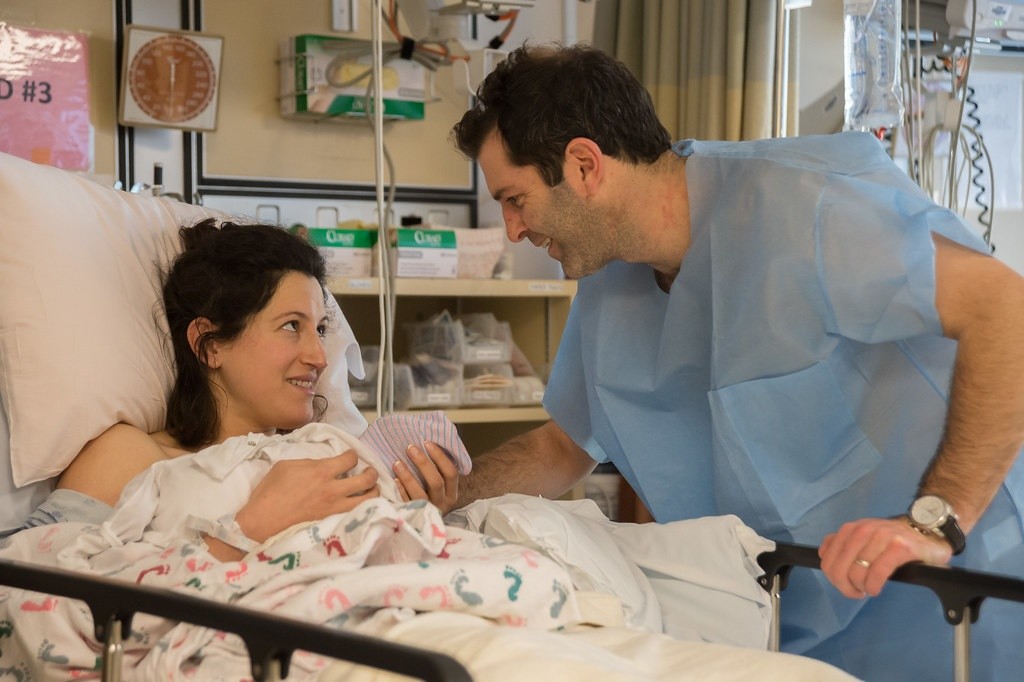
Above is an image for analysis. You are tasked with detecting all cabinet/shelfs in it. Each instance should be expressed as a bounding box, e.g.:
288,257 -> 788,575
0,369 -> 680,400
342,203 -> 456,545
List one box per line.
326,279 -> 579,502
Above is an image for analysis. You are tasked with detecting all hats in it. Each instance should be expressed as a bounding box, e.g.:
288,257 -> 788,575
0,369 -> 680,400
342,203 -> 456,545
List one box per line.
357,410 -> 472,481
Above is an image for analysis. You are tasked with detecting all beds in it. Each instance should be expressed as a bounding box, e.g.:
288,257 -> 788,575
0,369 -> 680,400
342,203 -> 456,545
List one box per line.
0,409 -> 1023,682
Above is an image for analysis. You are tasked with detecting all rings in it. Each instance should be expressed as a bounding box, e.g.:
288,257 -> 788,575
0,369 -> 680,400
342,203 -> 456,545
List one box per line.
854,557 -> 870,568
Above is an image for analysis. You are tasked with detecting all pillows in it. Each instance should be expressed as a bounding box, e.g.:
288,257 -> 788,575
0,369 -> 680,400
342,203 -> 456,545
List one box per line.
0,150 -> 369,489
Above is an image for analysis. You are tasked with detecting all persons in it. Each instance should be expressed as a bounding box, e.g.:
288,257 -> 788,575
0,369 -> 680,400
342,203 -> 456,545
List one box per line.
13,219 -> 866,682
442,39 -> 1024,682
115,409 -> 472,548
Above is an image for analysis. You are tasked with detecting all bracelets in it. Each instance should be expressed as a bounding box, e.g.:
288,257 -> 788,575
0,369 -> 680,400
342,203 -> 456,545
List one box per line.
188,511 -> 259,556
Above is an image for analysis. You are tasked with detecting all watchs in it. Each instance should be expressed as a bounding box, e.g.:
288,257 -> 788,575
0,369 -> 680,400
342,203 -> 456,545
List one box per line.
906,494 -> 967,557
